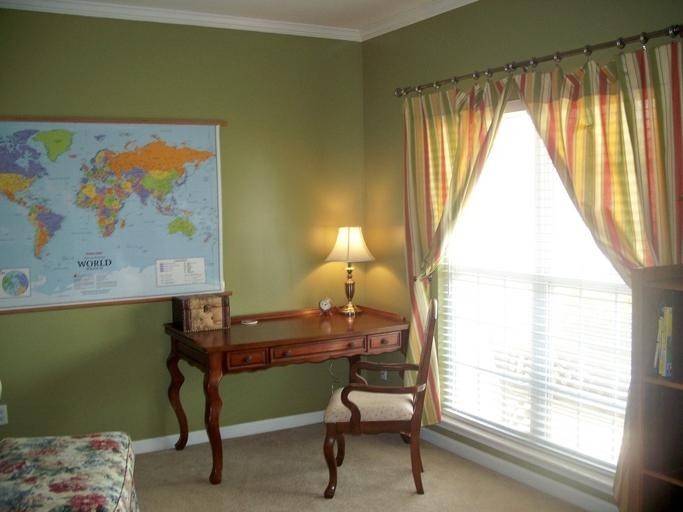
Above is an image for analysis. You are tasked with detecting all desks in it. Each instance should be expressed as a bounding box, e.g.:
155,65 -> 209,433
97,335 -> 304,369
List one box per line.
162,304 -> 411,486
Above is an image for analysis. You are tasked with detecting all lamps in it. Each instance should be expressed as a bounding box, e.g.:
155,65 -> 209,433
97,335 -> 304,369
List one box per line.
325,228 -> 376,315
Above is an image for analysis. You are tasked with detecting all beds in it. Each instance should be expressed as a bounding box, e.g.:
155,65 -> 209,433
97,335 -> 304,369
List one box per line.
0,431 -> 135,511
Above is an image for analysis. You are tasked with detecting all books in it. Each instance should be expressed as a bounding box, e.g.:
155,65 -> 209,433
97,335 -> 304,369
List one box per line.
651,303 -> 675,378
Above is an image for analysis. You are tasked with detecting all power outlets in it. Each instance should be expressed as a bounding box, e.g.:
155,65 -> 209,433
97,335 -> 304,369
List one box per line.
0,405 -> 8,427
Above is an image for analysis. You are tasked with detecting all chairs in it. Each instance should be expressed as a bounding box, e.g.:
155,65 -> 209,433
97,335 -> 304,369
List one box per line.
321,300 -> 439,502
